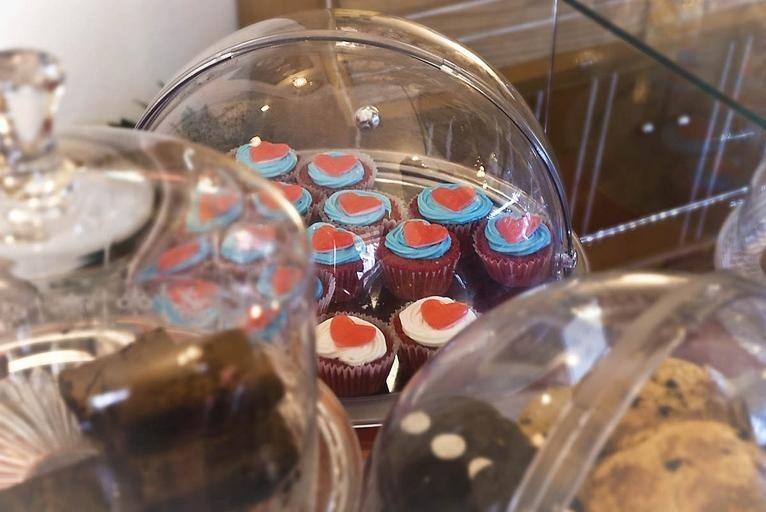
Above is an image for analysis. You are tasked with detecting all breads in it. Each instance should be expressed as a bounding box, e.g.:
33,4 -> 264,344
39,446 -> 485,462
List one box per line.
377,360 -> 764,512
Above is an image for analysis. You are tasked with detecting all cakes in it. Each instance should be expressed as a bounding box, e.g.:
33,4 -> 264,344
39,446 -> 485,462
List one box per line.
49,329 -> 300,512
125,176 -> 309,341
318,190 -> 399,228
296,150 -> 381,192
306,222 -> 365,302
390,295 -> 482,372
372,219 -> 462,298
475,209 -> 553,285
411,182 -> 492,223
226,136 -> 300,180
312,314 -> 393,395
